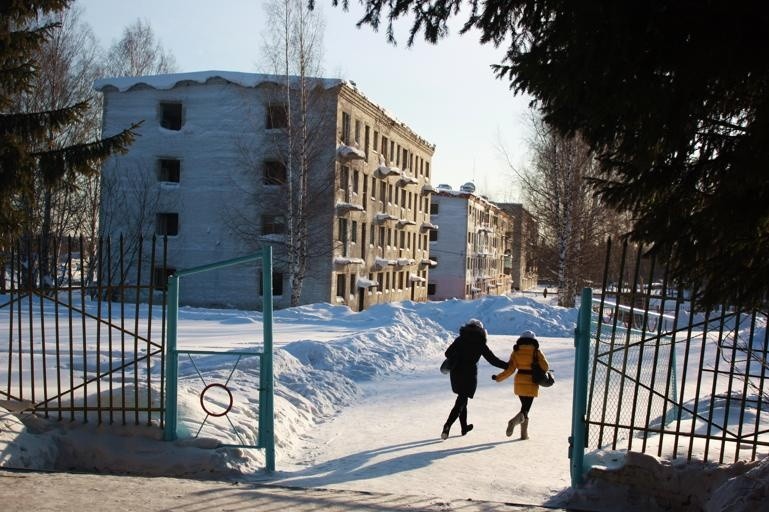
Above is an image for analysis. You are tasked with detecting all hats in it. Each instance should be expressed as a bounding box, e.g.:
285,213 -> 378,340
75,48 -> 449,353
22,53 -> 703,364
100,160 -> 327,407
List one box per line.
468,319 -> 483,328
520,330 -> 536,339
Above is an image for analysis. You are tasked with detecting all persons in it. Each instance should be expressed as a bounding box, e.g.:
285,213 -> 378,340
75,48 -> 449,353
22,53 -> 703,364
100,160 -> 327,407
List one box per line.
492,330 -> 549,439
441,319 -> 509,439
543,286 -> 547,298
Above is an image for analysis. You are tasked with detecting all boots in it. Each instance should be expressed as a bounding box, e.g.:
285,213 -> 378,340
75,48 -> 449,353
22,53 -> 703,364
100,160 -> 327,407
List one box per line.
440,408 -> 459,439
459,408 -> 473,434
506,411 -> 525,437
521,419 -> 530,440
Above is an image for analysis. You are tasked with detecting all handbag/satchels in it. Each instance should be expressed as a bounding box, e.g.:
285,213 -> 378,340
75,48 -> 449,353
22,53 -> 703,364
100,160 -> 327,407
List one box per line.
531,352 -> 555,387
